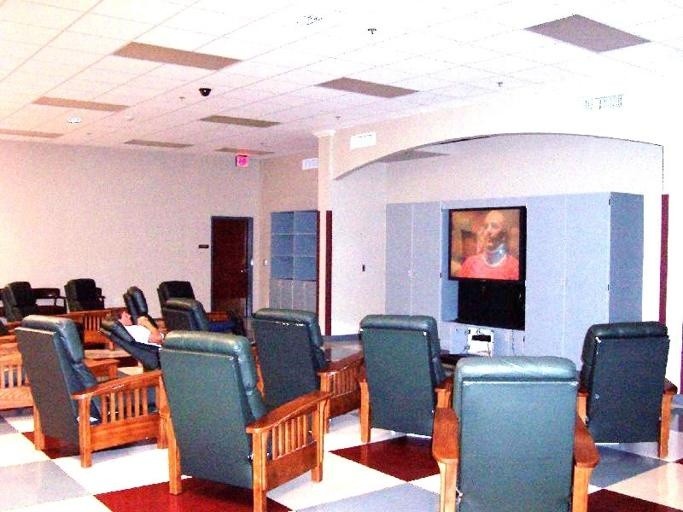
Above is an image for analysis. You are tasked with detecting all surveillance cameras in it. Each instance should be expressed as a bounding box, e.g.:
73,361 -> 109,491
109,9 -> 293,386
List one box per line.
200,88 -> 212,96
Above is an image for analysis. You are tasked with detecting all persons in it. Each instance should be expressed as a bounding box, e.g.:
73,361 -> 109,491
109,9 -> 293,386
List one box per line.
109,308 -> 166,349
456,211 -> 519,279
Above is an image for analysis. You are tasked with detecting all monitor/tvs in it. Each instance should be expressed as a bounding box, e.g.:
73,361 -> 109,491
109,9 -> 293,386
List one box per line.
448,206 -> 527,283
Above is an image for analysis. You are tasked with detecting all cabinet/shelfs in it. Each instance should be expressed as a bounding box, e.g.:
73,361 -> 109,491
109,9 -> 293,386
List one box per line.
268,209 -> 319,312
